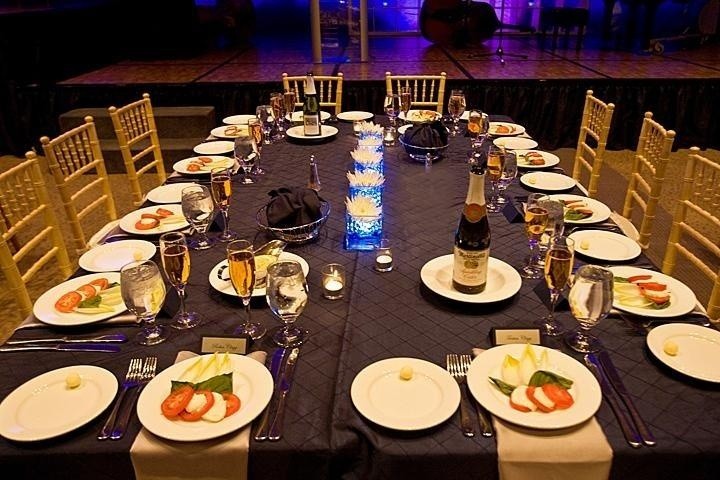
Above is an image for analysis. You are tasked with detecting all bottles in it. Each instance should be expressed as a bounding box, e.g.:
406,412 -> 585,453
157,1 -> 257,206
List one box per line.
303,72 -> 322,137
308,156 -> 320,191
452,166 -> 491,295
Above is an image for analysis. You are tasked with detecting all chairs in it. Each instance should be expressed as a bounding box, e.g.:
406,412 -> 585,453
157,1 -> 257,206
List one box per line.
0,151 -> 72,321
40,116 -> 117,257
624,111 -> 676,254
281,71 -> 344,118
661,146 -> 720,326
571,89 -> 616,202
109,93 -> 166,205
387,71 -> 446,118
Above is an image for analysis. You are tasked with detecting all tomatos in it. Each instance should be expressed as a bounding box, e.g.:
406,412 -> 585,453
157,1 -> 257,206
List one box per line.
509,396 -> 530,412
157,208 -> 173,215
135,219 -> 158,229
141,214 -> 166,219
78,284 -> 96,300
179,389 -> 212,420
56,291 -> 81,312
199,157 -> 212,163
89,278 -> 108,291
651,297 -> 669,304
637,283 -> 667,291
187,164 -> 199,171
627,275 -> 652,281
526,384 -> 555,411
221,391 -> 240,416
542,383 -> 573,407
160,384 -> 193,419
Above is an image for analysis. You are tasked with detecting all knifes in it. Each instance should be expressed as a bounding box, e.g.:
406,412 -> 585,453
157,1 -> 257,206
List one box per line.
253,347 -> 287,441
6,333 -> 128,343
0,343 -> 123,353
598,350 -> 656,447
583,353 -> 642,448
267,347 -> 301,440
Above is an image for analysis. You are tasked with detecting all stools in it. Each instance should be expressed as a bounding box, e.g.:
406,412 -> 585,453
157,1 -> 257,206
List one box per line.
550,6 -> 587,51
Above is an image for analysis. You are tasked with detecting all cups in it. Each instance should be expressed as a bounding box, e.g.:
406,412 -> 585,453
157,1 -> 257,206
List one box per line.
401,87 -> 412,128
119,259 -> 171,347
181,184 -> 215,252
282,87 -> 296,129
382,128 -> 396,147
353,120 -> 366,136
494,151 -> 517,204
374,239 -> 393,273
531,249 -> 575,337
566,264 -> 613,356
211,167 -> 238,244
448,89 -> 467,138
246,118 -> 265,175
159,231 -> 202,332
550,236 -> 574,277
286,124 -> 339,139
482,113 -> 489,143
271,91 -> 286,139
520,192 -> 552,280
468,110 -> 482,151
321,264 -> 346,300
485,148 -> 505,211
225,240 -> 268,342
383,94 -> 402,132
264,260 -> 309,350
541,199 -> 566,269
233,135 -> 257,186
255,104 -> 276,153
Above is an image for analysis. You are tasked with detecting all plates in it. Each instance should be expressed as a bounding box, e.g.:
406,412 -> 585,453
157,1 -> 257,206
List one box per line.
336,110 -> 374,124
78,238 -> 157,273
396,110 -> 443,125
119,203 -> 191,236
146,182 -> 201,205
172,155 -> 236,176
208,252 -> 311,300
646,321 -> 718,385
492,136 -> 539,151
193,139 -> 236,155
445,110 -> 489,121
486,122 -> 526,138
135,352 -> 275,444
0,363 -> 120,444
564,229 -> 642,262
519,171 -> 577,193
419,253 -> 522,304
210,124 -> 253,139
466,343 -> 604,432
222,114 -> 259,126
32,271 -> 129,327
349,356 -> 462,435
604,265 -> 697,319
510,149 -> 561,169
535,194 -> 612,226
284,110 -> 331,126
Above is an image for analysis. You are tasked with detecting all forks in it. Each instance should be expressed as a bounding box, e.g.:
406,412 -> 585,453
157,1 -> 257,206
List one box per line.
561,225 -> 619,234
96,357 -> 146,441
623,324 -> 710,338
445,353 -> 476,437
111,356 -> 159,439
618,314 -> 711,330
459,354 -> 494,437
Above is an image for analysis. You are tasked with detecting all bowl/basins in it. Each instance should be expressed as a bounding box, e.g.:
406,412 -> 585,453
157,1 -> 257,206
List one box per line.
255,196 -> 332,242
398,136 -> 451,162
